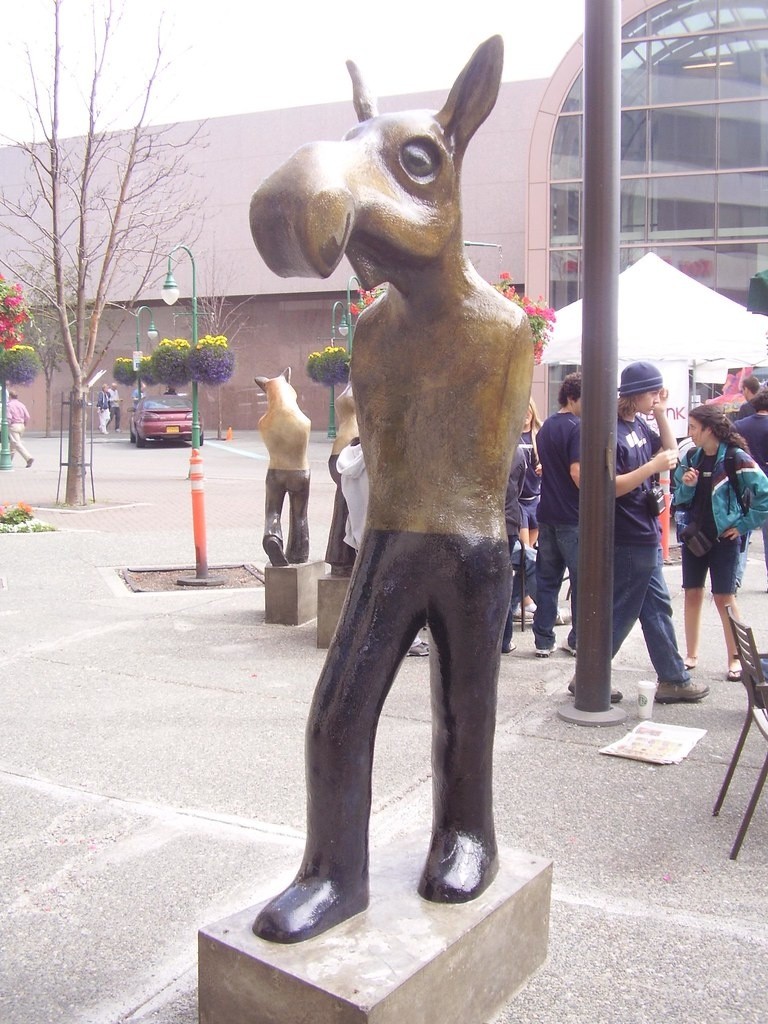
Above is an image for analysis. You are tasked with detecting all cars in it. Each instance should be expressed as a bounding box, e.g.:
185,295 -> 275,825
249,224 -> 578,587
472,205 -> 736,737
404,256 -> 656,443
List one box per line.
128,389 -> 205,448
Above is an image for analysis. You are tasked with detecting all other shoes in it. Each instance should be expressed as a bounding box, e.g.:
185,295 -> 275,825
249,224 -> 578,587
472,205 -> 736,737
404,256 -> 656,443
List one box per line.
563,642 -> 577,655
510,642 -> 516,650
512,609 -> 534,621
26,458 -> 33,467
407,642 -> 429,656
102,430 -> 109,434
116,429 -> 121,432
535,643 -> 557,656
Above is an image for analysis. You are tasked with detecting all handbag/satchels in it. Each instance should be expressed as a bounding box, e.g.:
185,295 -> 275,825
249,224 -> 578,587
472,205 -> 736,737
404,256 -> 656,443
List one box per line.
680,531 -> 713,557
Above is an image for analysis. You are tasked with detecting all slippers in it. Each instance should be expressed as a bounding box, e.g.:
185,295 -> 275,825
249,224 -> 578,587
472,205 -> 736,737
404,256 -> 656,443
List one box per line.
682,663 -> 696,670
727,670 -> 743,681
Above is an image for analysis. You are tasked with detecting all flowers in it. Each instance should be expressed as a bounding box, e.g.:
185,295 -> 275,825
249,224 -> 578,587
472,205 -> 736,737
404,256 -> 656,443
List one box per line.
140,356 -> 162,388
1,274 -> 35,350
491,273 -> 556,366
189,335 -> 236,385
112,357 -> 137,387
0,346 -> 42,387
308,345 -> 349,388
152,338 -> 192,389
350,281 -> 389,315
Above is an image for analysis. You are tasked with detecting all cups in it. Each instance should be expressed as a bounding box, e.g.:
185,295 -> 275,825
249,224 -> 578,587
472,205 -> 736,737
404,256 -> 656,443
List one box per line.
637,681 -> 657,719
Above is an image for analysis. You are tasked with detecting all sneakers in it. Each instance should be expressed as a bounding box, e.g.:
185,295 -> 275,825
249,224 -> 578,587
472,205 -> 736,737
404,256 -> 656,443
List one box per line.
655,681 -> 710,703
568,677 -> 623,702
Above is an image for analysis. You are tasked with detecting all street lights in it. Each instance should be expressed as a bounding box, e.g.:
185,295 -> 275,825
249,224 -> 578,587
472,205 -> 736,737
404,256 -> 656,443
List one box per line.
135,306 -> 159,399
327,276 -> 362,439
161,245 -> 224,585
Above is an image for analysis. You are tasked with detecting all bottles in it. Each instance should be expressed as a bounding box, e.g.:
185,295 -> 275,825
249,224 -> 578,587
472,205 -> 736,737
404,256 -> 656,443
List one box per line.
676,509 -> 687,544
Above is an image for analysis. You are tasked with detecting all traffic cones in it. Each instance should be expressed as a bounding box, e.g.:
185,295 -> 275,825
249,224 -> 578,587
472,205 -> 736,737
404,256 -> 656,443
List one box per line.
225,425 -> 232,440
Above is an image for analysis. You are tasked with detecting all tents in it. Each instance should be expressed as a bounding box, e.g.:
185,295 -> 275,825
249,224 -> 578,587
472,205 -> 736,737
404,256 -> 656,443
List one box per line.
523,252 -> 768,412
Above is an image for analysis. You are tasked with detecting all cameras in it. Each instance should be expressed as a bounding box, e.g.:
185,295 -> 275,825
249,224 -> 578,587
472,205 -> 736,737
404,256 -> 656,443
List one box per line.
647,486 -> 666,516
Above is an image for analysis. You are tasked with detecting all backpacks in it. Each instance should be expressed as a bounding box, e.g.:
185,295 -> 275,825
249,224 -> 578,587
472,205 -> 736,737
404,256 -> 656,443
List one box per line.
687,447 -> 752,553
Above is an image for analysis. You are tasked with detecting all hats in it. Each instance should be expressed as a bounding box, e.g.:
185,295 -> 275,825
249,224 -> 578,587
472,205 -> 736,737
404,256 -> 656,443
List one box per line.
619,363 -> 663,395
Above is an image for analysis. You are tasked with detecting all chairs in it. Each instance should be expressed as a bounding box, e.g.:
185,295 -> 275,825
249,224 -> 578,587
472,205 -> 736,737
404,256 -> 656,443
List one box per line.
711,605 -> 768,857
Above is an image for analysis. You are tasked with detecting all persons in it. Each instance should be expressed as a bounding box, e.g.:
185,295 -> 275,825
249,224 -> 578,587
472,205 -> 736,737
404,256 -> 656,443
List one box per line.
6,391 -> 34,468
501,396 -> 572,653
533,372 -> 582,656
337,436 -> 430,657
569,360 -> 711,703
131,381 -> 146,407
105,383 -> 123,433
164,388 -> 177,395
727,375 -> 768,594
673,404 -> 768,680
96,384 -> 110,435
